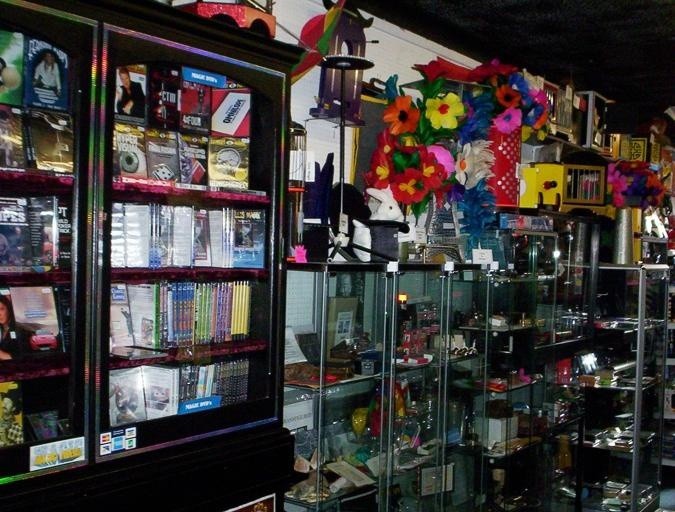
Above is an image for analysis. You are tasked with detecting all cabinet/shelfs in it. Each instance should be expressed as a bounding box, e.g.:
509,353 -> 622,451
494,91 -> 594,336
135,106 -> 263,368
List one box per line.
1,0 -> 294,511
283,249 -> 673,509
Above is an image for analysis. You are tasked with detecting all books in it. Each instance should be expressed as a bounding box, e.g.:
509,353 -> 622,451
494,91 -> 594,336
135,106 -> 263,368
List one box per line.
1,31 -> 78,444
107,64 -> 266,428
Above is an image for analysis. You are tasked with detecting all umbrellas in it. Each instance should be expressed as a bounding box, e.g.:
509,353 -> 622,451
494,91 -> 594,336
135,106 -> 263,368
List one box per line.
245,0 -> 348,87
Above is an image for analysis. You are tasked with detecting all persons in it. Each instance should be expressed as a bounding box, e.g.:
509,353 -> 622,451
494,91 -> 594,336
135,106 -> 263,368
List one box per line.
235,223 -> 253,247
116,67 -> 145,117
31,50 -> 62,96
2,295 -> 24,360
